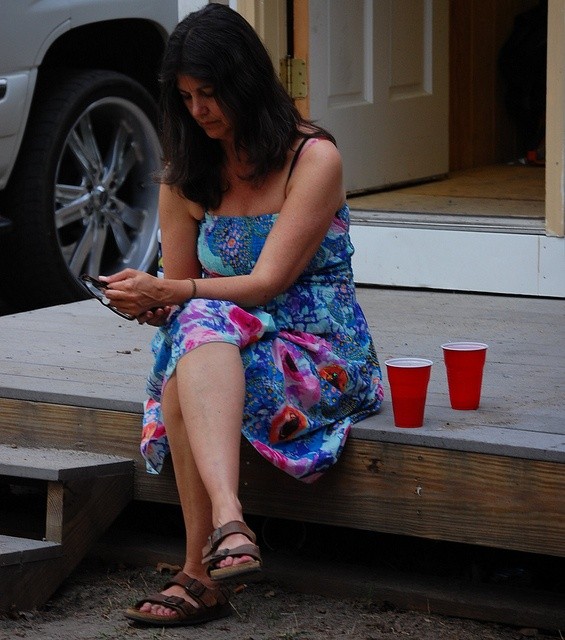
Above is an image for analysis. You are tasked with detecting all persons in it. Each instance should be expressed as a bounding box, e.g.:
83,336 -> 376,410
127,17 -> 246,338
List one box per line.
98,0 -> 386,624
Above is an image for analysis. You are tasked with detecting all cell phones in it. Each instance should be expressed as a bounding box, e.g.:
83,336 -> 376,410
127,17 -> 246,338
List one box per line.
78,276 -> 164,320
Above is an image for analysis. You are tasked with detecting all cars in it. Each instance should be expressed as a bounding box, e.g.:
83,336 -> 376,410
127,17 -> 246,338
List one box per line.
0,3 -> 190,311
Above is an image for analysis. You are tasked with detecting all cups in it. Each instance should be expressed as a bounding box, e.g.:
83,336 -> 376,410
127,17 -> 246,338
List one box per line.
441,341 -> 488,410
384,358 -> 434,429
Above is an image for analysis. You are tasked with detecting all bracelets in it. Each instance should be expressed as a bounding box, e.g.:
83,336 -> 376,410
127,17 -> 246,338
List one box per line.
186,278 -> 198,301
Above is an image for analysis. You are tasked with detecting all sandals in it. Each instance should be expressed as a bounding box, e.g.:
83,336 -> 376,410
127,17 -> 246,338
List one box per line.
124,571 -> 230,627
200,520 -> 262,581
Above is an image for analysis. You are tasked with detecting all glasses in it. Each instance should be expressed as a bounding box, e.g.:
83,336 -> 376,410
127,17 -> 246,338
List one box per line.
78,273 -> 138,321
